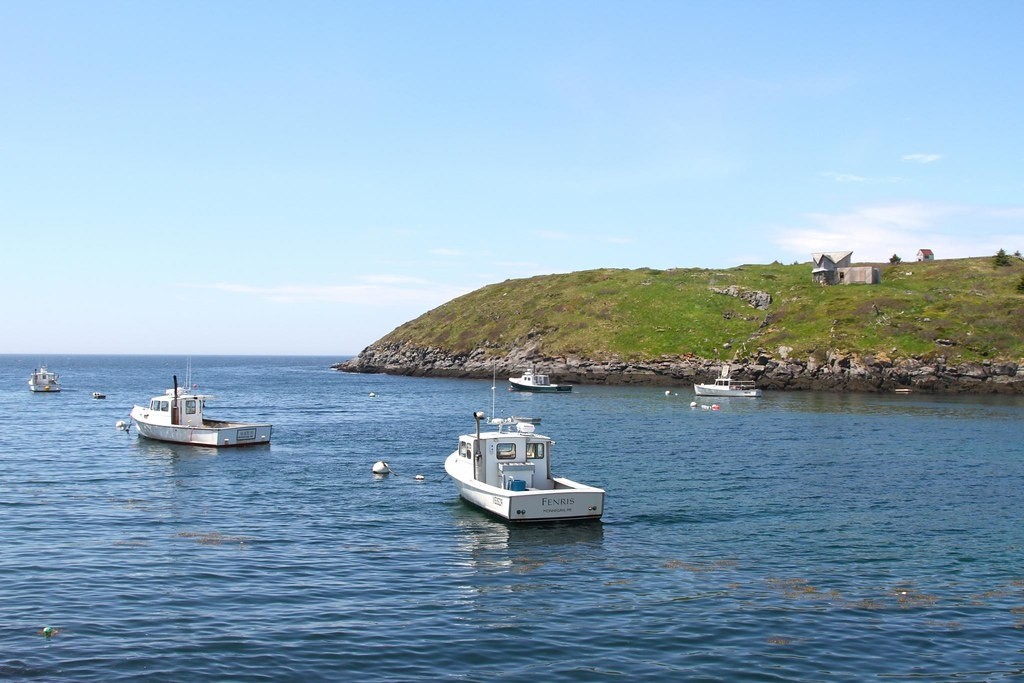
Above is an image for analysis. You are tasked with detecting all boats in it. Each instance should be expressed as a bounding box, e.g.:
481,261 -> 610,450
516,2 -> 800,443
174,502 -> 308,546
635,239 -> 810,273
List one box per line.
693,364 -> 762,398
28,365 -> 61,393
508,368 -> 573,393
127,356 -> 274,448
443,358 -> 607,525
